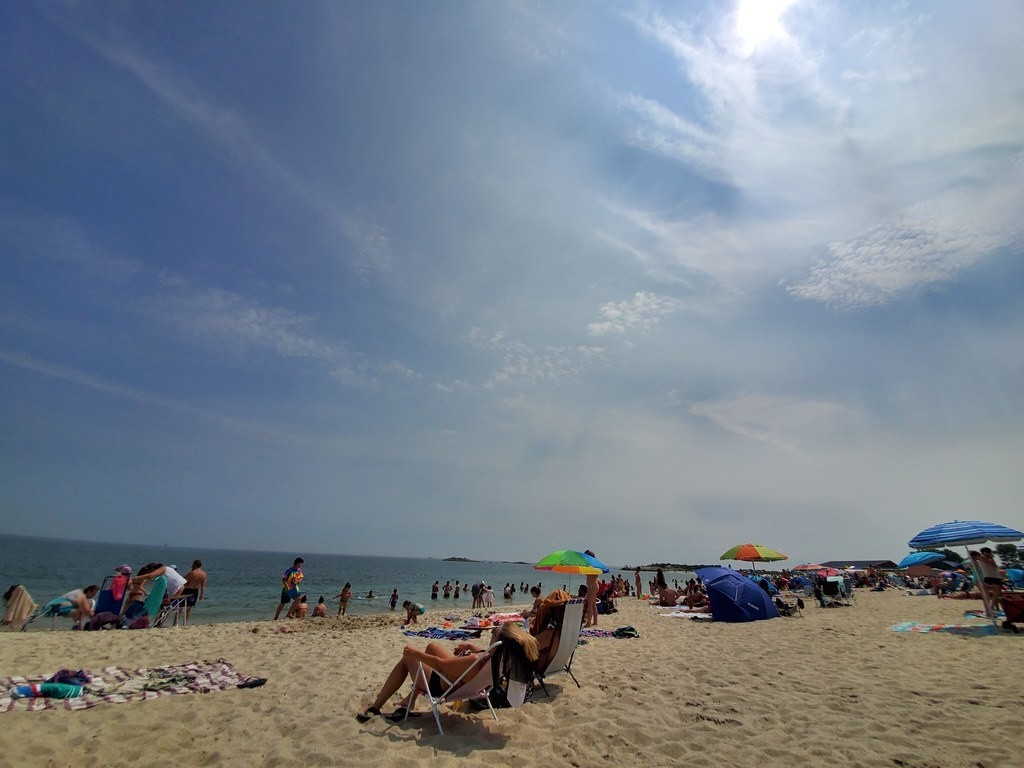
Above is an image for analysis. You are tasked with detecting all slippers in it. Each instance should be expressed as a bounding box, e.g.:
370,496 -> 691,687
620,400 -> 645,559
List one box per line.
358,707 -> 388,723
237,676 -> 268,689
385,708 -> 422,721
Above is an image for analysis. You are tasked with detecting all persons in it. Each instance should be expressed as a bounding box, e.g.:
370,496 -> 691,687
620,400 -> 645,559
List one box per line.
43,559 -> 207,631
354,620 -> 540,723
528,589 -> 572,671
974,547 -> 1003,613
310,595 -> 327,617
332,581 -> 353,616
968,550 -> 997,618
431,563 -> 1007,619
366,590 -> 376,598
584,550 -> 602,628
402,600 -> 425,624
272,557 -> 305,620
295,595 -> 309,618
389,588 -> 399,610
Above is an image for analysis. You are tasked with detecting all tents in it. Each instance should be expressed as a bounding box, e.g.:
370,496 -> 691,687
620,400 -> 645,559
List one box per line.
694,566 -> 782,623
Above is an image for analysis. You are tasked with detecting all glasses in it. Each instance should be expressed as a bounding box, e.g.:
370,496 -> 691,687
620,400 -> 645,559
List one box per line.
491,627 -> 498,641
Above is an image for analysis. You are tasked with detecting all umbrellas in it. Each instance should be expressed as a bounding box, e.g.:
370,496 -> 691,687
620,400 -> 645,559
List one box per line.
907,518 -> 1024,629
896,550 -> 946,575
791,561 -> 828,572
533,549 -> 610,595
718,541 -> 789,583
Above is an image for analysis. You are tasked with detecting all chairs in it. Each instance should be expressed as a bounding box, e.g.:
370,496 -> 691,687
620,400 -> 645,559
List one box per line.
842,579 -> 856,604
525,595 -> 589,700
812,587 -> 840,608
776,598 -> 805,618
940,579 -> 962,595
404,636 -> 534,736
3,585 -> 61,632
823,581 -> 850,607
983,576 -> 1024,632
92,575 -> 145,629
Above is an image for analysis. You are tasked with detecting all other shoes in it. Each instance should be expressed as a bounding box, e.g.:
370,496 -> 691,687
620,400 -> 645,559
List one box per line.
493,620 -> 502,626
474,611 -> 497,617
